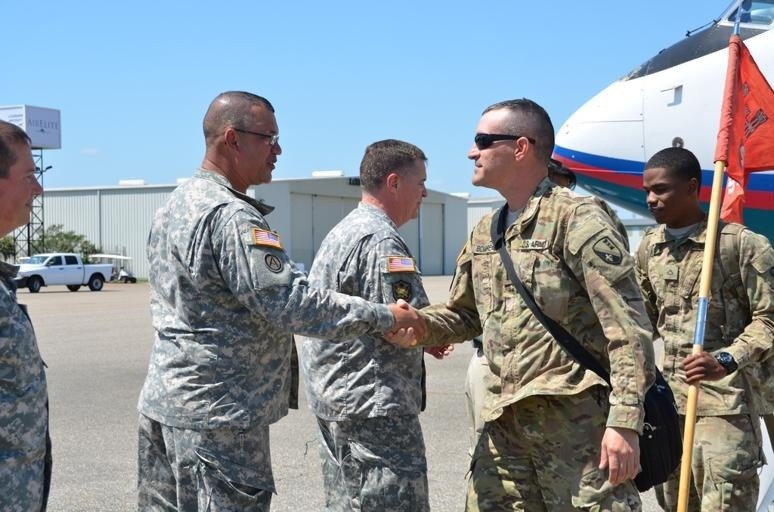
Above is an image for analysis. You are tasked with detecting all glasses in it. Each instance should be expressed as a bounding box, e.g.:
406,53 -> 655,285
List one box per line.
234,128 -> 280,146
475,133 -> 535,150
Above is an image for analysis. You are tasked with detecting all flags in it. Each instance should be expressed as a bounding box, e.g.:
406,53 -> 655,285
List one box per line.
716,36 -> 774,223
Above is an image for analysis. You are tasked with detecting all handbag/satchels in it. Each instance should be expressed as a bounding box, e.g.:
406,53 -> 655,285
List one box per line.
633,365 -> 682,493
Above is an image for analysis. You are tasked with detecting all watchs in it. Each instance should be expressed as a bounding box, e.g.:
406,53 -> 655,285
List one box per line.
716,352 -> 737,373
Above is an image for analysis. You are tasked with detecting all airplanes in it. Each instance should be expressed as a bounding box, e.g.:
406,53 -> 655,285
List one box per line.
548,0 -> 773,255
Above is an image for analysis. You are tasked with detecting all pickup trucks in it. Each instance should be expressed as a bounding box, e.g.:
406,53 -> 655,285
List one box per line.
12,251 -> 114,293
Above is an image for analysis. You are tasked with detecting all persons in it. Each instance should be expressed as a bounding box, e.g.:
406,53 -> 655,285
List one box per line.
134,90 -> 427,512
303,140 -> 453,512
0,120 -> 51,511
633,146 -> 758,512
384,99 -> 657,511
